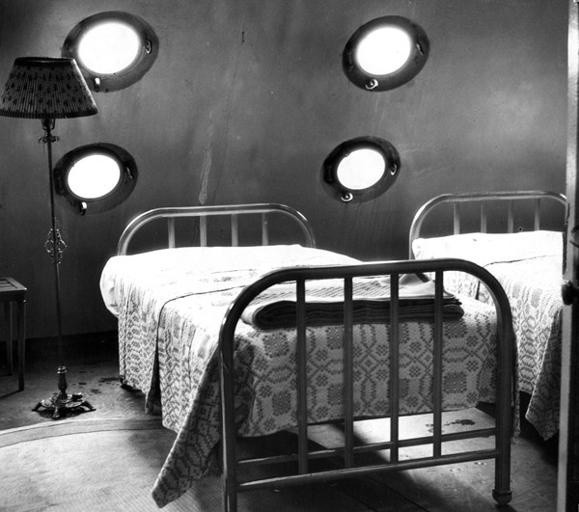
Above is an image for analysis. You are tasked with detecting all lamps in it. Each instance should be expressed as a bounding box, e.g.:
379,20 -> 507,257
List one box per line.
1,54 -> 100,422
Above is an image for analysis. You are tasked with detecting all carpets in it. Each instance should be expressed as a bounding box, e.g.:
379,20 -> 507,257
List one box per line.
0,410 -> 432,512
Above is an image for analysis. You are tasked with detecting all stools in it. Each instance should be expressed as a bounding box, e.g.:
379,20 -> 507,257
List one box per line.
1,274 -> 33,393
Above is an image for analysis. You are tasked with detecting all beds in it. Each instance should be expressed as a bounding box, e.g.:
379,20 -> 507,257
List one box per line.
98,199 -> 523,511
406,188 -> 568,444
